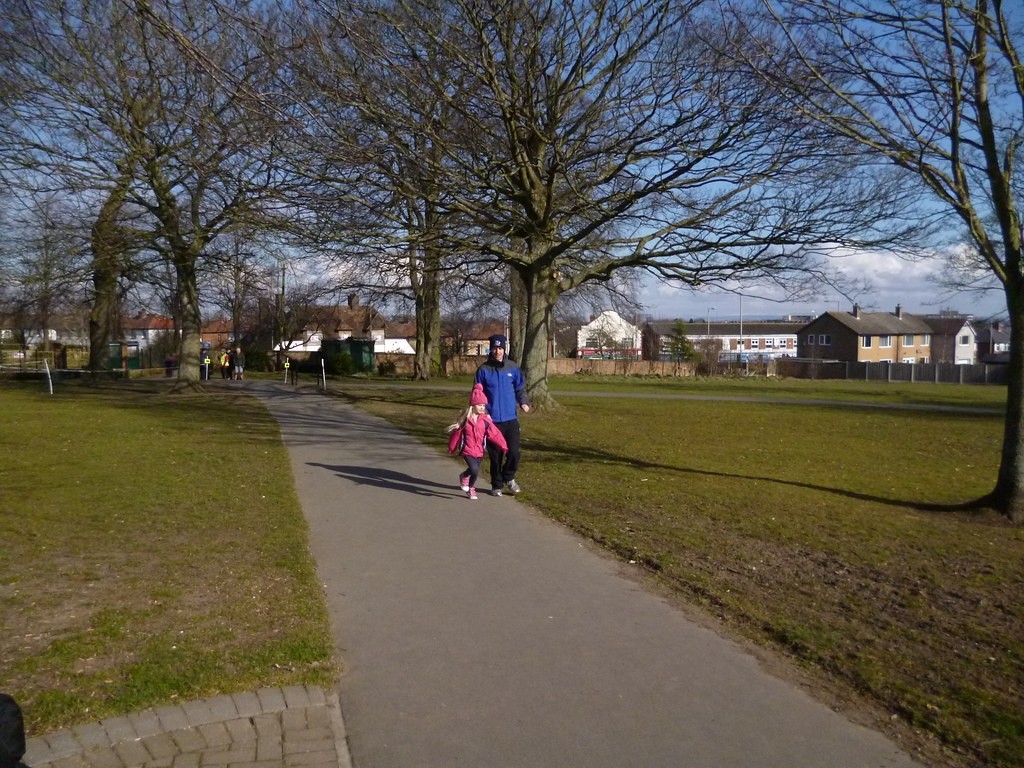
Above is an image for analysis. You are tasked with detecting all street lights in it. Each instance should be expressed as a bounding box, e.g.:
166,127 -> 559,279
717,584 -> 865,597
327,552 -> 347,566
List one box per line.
824,300 -> 839,311
707,307 -> 716,335
740,293 -> 744,368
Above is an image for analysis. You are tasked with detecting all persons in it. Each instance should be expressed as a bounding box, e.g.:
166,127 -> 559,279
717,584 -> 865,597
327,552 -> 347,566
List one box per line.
201,348 -> 245,381
473,334 -> 530,496
444,383 -> 509,499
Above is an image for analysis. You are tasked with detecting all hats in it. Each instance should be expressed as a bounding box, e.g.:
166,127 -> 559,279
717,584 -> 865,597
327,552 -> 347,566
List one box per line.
470,383 -> 488,405
488,334 -> 508,350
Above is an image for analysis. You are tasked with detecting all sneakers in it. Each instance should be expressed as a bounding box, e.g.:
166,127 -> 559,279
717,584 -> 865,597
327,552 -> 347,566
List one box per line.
469,487 -> 478,500
459,472 -> 470,492
503,479 -> 521,494
491,487 -> 502,497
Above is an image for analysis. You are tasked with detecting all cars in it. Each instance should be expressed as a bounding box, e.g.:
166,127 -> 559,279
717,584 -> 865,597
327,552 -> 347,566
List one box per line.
2,344 -> 32,359
